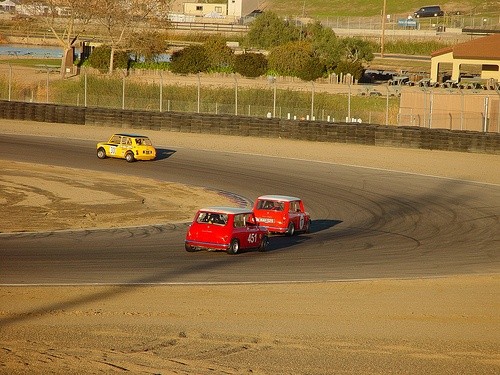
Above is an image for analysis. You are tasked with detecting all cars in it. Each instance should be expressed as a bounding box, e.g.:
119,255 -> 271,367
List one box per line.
96,133 -> 156,162
185,207 -> 270,255
247,194 -> 311,237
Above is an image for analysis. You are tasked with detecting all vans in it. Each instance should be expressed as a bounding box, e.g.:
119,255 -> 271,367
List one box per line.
414,6 -> 441,18
